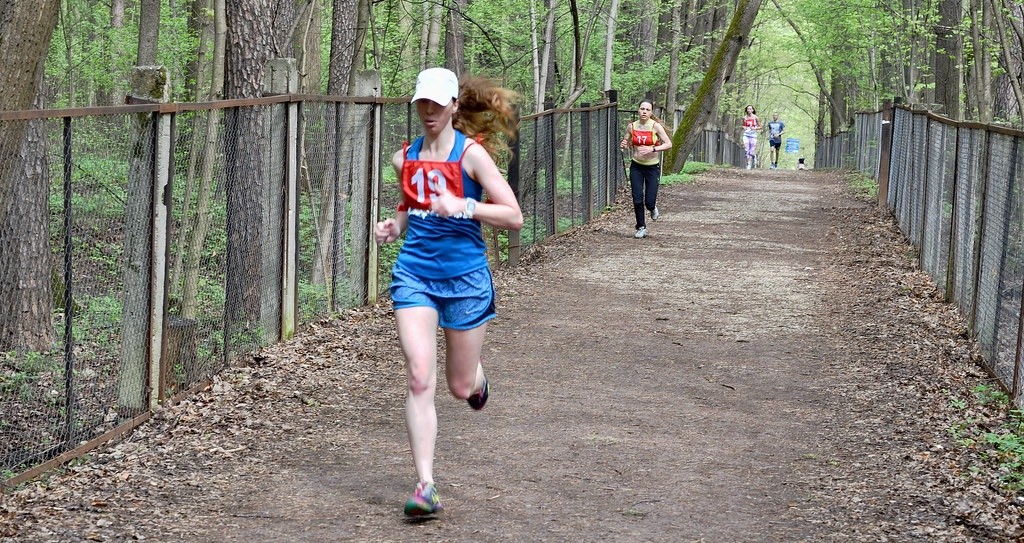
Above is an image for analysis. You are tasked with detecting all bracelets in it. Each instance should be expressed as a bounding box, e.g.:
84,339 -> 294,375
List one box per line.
652,146 -> 656,152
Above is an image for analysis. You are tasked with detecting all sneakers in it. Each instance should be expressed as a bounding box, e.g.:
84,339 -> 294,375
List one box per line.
634,226 -> 649,239
404,481 -> 444,516
754,165 -> 757,170
651,206 -> 659,220
747,165 -> 751,170
466,359 -> 489,410
770,164 -> 778,169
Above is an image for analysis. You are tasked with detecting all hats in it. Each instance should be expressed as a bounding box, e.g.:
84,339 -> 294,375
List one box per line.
410,68 -> 459,106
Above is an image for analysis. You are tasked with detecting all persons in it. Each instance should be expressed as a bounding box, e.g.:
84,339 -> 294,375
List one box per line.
767,112 -> 786,169
374,67 -> 523,518
620,99 -> 673,238
799,155 -> 805,170
739,105 -> 762,169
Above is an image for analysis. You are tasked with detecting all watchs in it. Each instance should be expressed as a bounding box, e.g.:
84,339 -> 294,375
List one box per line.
464,197 -> 477,218
